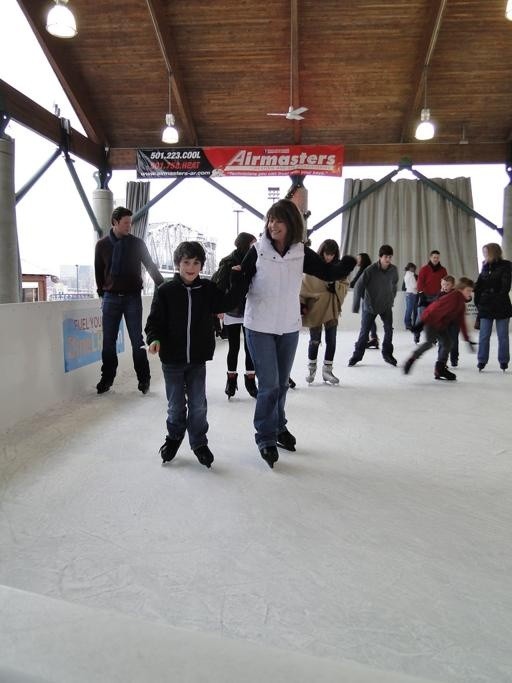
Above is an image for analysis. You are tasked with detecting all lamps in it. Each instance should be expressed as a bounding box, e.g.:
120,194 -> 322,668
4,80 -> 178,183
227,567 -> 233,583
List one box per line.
43,0 -> 78,38
160,72 -> 179,144
414,64 -> 436,141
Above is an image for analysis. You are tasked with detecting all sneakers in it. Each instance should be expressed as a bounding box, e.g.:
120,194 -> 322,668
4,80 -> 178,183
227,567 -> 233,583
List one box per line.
304,365 -> 316,383
281,419 -> 295,446
188,443 -> 216,466
160,436 -> 183,462
225,373 -> 239,396
434,361 -> 456,379
322,365 -> 339,384
95,373 -> 115,389
413,332 -> 421,341
350,356 -> 362,366
366,339 -> 377,348
138,376 -> 151,392
262,444 -> 281,465
404,358 -> 413,374
244,373 -> 258,396
383,352 -> 397,364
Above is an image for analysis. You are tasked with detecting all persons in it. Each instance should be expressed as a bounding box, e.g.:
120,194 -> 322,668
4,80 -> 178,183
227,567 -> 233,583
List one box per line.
145,232 -> 257,468
474,243 -> 512,373
402,250 -> 477,380
95,207 -> 166,394
227,200 -> 358,468
348,245 -> 398,367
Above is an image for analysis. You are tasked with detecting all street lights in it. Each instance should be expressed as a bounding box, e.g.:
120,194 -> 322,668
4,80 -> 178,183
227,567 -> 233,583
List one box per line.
267,185 -> 281,204
234,209 -> 244,239
75,264 -> 79,296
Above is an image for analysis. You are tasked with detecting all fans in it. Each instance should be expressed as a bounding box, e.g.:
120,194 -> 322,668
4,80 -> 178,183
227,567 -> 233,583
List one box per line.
265,104 -> 310,122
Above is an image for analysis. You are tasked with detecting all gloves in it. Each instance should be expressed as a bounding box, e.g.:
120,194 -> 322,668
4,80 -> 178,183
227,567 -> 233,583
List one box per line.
411,322 -> 424,333
